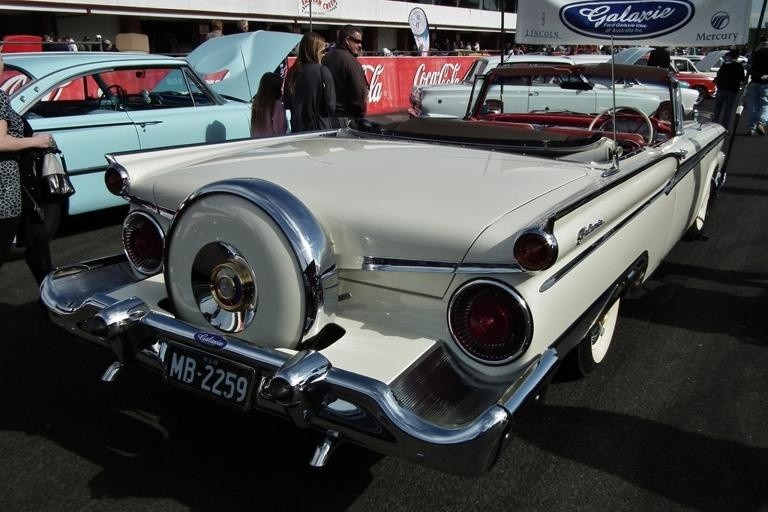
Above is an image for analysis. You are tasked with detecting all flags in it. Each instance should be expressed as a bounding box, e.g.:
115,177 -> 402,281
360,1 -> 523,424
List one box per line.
408,7 -> 430,56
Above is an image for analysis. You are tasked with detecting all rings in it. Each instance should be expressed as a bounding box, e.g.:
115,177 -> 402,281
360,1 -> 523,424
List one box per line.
50,138 -> 52,142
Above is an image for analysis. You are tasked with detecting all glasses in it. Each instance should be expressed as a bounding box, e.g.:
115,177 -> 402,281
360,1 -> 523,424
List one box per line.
350,35 -> 362,44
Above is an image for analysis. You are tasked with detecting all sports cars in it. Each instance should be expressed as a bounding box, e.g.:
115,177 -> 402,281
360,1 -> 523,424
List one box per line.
39,31 -> 729,469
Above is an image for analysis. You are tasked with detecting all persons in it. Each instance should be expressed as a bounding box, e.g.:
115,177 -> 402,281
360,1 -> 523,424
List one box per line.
378,48 -> 404,57
743,41 -> 768,137
712,50 -> 744,130
358,48 -> 364,55
206,19 -> 224,40
430,40 -> 480,56
41,32 -> 117,51
247,73 -> 288,140
233,19 -> 248,34
0,46 -> 58,317
283,32 -> 335,131
322,25 -> 370,124
505,43 -> 629,57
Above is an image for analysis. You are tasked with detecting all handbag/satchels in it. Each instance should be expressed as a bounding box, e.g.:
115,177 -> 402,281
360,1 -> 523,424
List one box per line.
35,195 -> 71,235
312,113 -> 335,130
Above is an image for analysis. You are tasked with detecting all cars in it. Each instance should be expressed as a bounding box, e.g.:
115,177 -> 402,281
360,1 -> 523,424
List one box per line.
1,30 -> 307,224
408,45 -> 729,118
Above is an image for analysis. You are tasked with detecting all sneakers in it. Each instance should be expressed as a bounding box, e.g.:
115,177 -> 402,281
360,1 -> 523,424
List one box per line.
745,128 -> 755,135
757,125 -> 766,135
